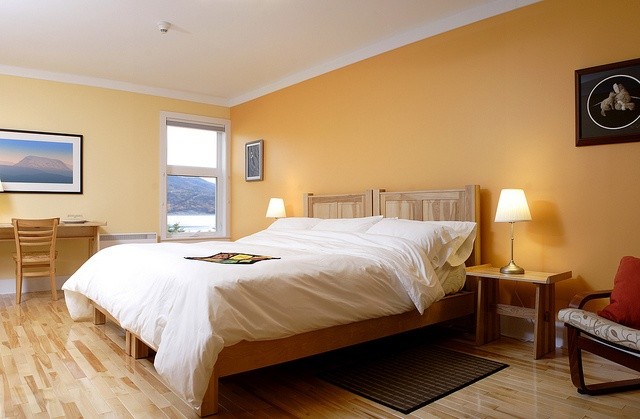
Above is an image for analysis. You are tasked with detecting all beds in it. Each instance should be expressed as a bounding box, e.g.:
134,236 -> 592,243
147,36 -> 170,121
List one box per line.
91,184 -> 498,417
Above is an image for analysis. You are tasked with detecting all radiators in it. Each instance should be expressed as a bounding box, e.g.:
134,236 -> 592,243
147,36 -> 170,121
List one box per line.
93,232 -> 158,251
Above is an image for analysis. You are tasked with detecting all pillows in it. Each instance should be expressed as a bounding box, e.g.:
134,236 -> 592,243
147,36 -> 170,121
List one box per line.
598,255 -> 640,329
312,215 -> 383,234
367,217 -> 458,262
418,219 -> 478,267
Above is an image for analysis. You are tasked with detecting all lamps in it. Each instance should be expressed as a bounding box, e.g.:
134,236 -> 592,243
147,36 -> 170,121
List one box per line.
494,189 -> 533,275
265,197 -> 286,219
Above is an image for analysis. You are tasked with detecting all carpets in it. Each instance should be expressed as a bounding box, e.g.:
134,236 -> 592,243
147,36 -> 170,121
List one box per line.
314,345 -> 511,415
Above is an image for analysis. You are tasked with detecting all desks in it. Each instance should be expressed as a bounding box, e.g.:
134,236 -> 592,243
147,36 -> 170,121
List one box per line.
0,217 -> 108,254
463,267 -> 573,360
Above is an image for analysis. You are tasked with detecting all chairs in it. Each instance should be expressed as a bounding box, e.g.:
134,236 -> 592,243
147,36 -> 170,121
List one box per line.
11,218 -> 60,304
556,289 -> 639,396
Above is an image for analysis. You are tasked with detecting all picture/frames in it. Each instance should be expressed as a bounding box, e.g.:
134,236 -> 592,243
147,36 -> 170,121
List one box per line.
245,140 -> 263,181
574,58 -> 640,147
0,129 -> 83,194
